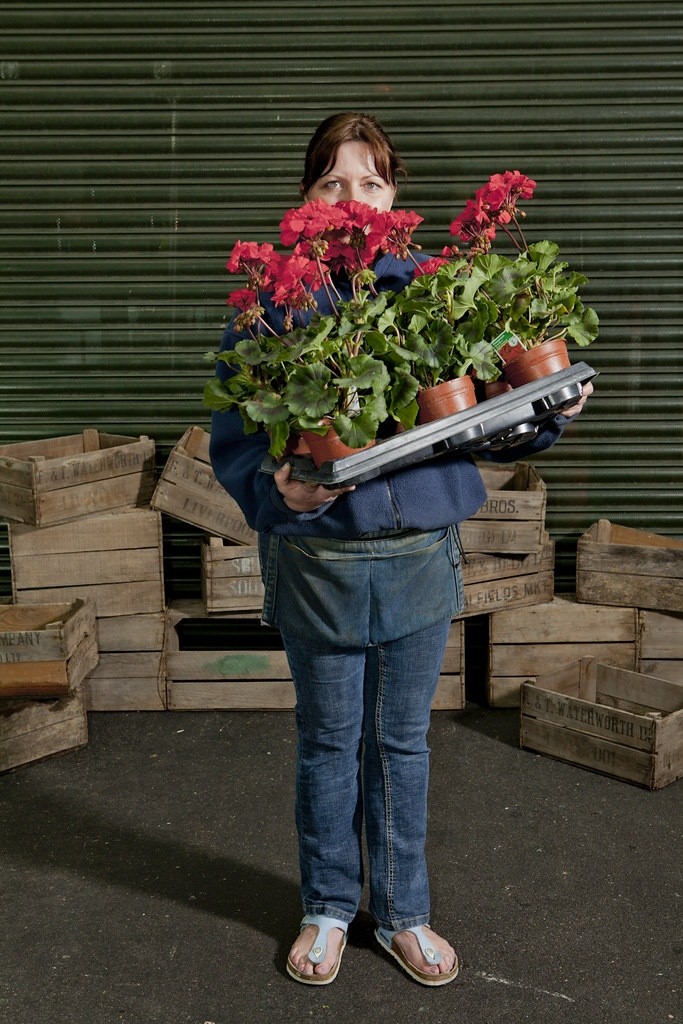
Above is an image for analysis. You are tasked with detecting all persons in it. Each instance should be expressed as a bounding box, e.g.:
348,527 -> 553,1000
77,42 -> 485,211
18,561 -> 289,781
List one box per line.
207,107 -> 596,990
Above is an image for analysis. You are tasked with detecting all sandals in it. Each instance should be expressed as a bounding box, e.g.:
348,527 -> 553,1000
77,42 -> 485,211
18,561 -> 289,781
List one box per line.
286,914 -> 349,986
374,923 -> 459,987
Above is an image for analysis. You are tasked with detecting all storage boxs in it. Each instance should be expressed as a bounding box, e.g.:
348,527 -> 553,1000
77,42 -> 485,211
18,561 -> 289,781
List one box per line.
426,463 -> 683,791
0,428 -> 292,775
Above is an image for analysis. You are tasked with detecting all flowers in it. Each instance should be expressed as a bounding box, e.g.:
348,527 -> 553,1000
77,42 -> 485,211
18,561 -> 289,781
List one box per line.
411,168 -> 599,359
278,198 -> 463,380
210,238 -> 357,431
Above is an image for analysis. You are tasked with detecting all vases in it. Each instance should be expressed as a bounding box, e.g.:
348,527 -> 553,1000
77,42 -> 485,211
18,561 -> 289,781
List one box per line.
407,371 -> 481,427
292,405 -> 376,474
497,335 -> 571,392
